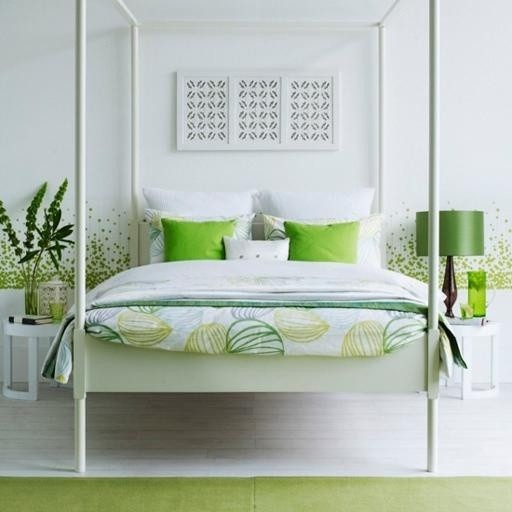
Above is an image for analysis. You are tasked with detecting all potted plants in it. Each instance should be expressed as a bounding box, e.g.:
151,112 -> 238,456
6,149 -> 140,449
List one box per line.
0,176 -> 75,316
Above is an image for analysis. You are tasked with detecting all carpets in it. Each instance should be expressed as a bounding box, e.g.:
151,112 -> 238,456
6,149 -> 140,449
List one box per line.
254,475 -> 512,512
0,474 -> 254,512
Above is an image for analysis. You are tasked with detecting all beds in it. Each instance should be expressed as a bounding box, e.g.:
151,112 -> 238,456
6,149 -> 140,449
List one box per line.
68,220 -> 451,475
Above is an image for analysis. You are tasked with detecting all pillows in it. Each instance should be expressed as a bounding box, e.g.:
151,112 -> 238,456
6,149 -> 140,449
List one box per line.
133,184 -> 386,272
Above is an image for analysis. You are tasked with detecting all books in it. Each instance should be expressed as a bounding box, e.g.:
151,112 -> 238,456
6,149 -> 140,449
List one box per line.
9,314 -> 54,325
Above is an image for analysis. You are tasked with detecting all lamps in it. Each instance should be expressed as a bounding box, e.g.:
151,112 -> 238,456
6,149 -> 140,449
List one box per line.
413,210 -> 484,320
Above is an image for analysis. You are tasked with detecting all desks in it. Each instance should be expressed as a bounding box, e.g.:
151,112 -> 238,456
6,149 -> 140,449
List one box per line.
2,319 -> 65,403
445,321 -> 498,401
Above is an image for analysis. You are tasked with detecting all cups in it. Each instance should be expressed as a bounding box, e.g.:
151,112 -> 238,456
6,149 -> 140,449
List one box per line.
49,301 -> 64,323
